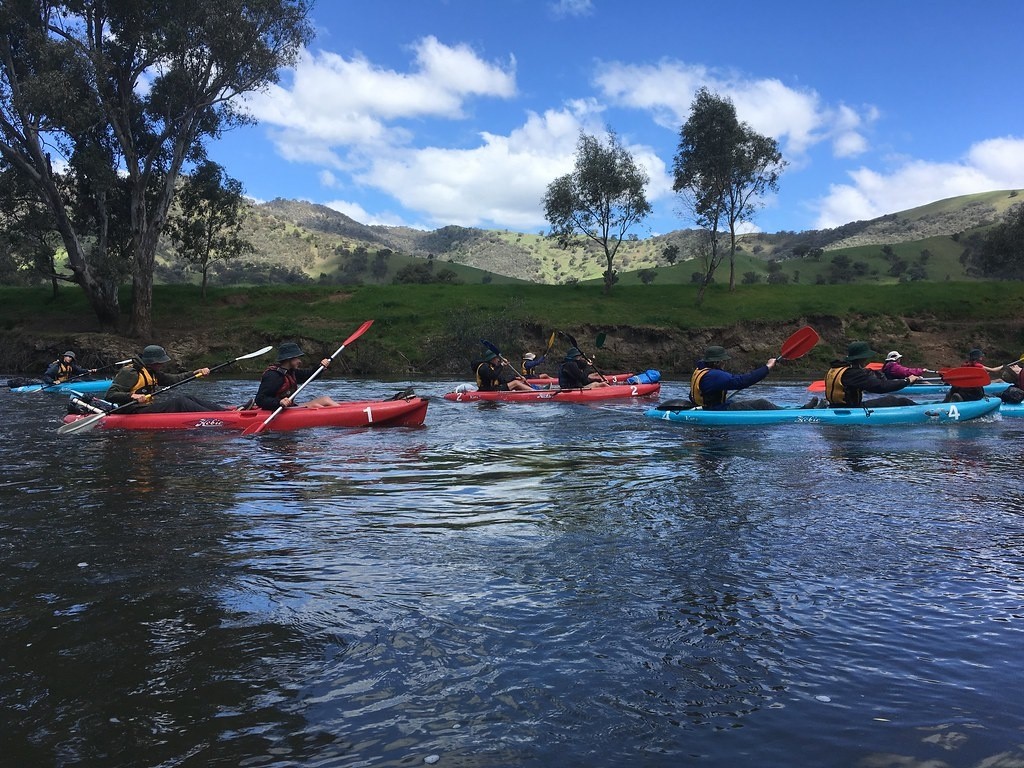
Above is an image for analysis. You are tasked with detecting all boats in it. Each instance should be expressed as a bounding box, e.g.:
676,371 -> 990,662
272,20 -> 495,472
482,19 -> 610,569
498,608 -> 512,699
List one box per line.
9,379 -> 116,392
642,371 -> 1024,425
443,371 -> 662,401
64,396 -> 432,431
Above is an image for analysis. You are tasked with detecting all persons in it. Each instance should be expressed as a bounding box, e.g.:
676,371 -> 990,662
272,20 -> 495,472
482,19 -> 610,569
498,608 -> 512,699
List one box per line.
255,342 -> 339,409
883,351 -> 932,384
557,348 -> 611,390
690,346 -> 818,410
476,349 -> 533,390
104,344 -> 255,412
825,341 -> 920,407
960,349 -> 1003,373
520,353 -> 551,379
42,351 -> 97,386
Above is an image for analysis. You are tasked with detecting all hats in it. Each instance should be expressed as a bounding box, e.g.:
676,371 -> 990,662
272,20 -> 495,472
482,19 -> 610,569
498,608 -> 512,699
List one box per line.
485,349 -> 501,361
702,345 -> 732,362
885,351 -> 902,361
141,345 -> 171,364
567,346 -> 584,358
969,349 -> 984,361
276,343 -> 306,362
523,352 -> 536,361
61,351 -> 76,361
846,341 -> 877,361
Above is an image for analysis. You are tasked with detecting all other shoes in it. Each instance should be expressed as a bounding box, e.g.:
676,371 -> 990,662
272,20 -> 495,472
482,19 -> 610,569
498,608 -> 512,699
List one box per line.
800,396 -> 819,409
237,398 -> 254,411
542,382 -> 553,389
815,399 -> 826,409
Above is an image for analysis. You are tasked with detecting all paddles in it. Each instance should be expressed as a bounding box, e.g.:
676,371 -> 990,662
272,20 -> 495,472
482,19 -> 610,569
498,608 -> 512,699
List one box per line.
564,332 -> 612,386
480,338 -> 531,387
58,344 -> 272,434
727,326 -> 821,399
241,318 -> 376,436
865,362 -> 956,375
589,330 -> 607,371
33,358 -> 133,394
807,366 -> 992,393
987,354 -> 1024,375
546,332 -> 555,355
